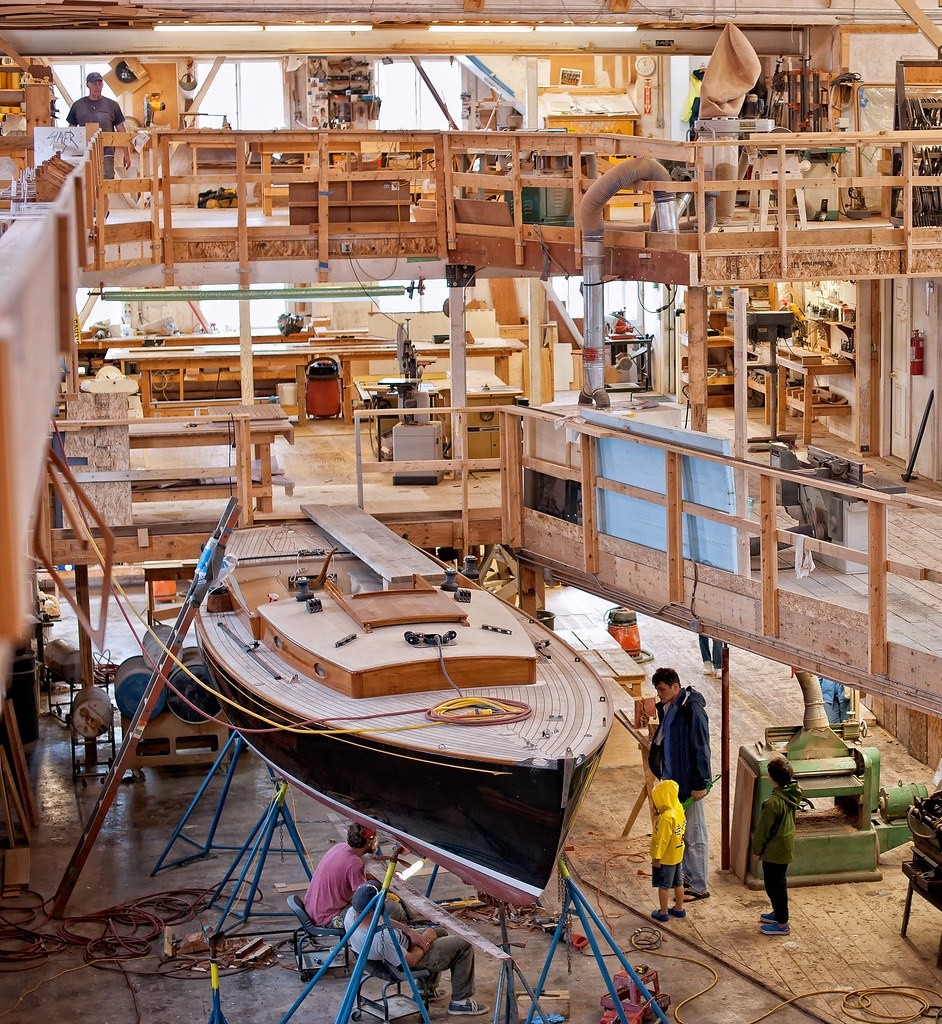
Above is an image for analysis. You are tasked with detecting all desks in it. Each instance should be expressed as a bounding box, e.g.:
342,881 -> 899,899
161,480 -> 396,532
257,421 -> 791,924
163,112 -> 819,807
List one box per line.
142,561 -> 198,627
129,420 -> 294,514
188,143 -> 436,217
104,338 -> 528,425
555,627 -> 646,698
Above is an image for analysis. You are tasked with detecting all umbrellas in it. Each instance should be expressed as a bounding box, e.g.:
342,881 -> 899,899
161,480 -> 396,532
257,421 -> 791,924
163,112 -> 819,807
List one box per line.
683,774 -> 722,808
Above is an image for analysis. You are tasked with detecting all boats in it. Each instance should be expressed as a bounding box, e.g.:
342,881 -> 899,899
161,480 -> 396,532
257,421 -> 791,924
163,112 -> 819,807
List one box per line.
193,501 -> 616,911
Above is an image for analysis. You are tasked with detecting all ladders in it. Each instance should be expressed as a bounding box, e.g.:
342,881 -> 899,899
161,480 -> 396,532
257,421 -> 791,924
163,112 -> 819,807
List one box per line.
49,495 -> 242,921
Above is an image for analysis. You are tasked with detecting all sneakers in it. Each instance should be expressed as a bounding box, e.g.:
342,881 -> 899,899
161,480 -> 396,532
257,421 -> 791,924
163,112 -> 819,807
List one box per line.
759,922 -> 791,935
702,661 -> 713,675
412,988 -> 448,1002
448,997 -> 490,1015
760,911 -> 777,924
714,669 -> 723,679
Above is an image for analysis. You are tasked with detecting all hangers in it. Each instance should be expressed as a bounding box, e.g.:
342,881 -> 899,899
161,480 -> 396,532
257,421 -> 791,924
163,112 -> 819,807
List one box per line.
697,62 -> 708,74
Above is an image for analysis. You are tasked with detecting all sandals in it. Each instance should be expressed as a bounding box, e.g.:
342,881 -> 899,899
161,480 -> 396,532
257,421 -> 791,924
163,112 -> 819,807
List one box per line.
651,909 -> 669,922
668,905 -> 686,917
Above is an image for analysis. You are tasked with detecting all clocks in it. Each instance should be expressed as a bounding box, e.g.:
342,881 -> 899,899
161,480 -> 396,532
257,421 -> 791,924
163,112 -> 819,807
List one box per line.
635,55 -> 655,77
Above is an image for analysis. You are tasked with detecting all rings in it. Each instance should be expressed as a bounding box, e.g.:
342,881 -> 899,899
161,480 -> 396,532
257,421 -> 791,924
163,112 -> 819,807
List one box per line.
427,942 -> 428,944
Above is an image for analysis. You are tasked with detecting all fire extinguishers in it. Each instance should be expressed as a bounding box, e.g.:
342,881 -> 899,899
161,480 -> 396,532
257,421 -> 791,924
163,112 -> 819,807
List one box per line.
910,328 -> 924,376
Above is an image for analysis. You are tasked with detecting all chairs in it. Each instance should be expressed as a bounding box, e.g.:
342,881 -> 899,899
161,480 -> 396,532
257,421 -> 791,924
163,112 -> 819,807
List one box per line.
350,950 -> 431,1024
288,895 -> 349,982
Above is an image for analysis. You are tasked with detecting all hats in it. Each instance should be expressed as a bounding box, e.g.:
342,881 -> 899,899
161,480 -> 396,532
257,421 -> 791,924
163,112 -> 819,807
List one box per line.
353,879 -> 382,914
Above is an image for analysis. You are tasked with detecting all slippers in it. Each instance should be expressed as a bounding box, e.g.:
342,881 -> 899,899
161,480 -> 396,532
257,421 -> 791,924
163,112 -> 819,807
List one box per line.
671,882 -> 691,888
674,890 -> 710,902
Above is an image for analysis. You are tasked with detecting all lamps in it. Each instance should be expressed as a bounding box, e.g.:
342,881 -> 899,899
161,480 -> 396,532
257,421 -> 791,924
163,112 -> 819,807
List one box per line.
426,23 -> 640,33
151,20 -> 376,32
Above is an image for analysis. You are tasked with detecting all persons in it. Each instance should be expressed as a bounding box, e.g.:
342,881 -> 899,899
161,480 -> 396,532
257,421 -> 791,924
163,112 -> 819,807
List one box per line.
648,668 -> 712,903
343,880 -> 489,1015
66,72 -> 132,224
699,635 -> 722,679
752,757 -> 803,934
649,780 -> 687,922
303,822 -> 403,928
817,678 -> 848,724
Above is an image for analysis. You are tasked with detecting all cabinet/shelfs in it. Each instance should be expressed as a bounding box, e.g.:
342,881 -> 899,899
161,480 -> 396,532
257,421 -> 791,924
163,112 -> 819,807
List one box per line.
777,359 -> 853,445
680,282 -> 776,386
0,88 -> 28,167
432,385 -> 523,474
